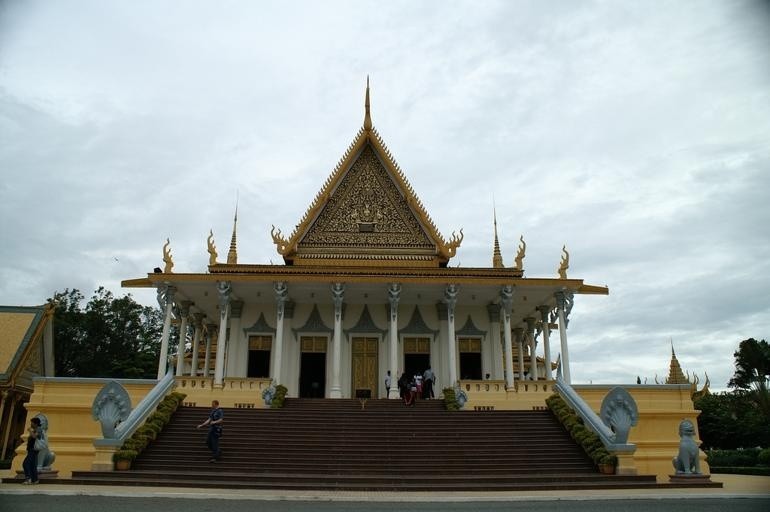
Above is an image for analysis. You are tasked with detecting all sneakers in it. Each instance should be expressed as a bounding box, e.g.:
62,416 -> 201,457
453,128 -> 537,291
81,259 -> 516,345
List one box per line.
209,450 -> 225,463
22,478 -> 40,485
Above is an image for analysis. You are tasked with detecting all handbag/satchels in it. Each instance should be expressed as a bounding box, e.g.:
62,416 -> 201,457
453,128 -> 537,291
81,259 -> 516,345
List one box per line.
213,425 -> 222,433
33,436 -> 46,451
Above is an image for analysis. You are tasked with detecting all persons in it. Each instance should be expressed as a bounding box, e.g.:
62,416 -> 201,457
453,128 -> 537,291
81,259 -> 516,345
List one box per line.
485,373 -> 491,380
385,365 -> 437,408
524,371 -> 532,380
636,375 -> 642,384
22,417 -> 43,485
197,399 -> 224,464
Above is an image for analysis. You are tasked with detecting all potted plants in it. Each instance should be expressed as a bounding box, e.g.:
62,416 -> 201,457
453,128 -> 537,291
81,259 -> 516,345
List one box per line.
544,391 -> 617,475
112,392 -> 187,471
